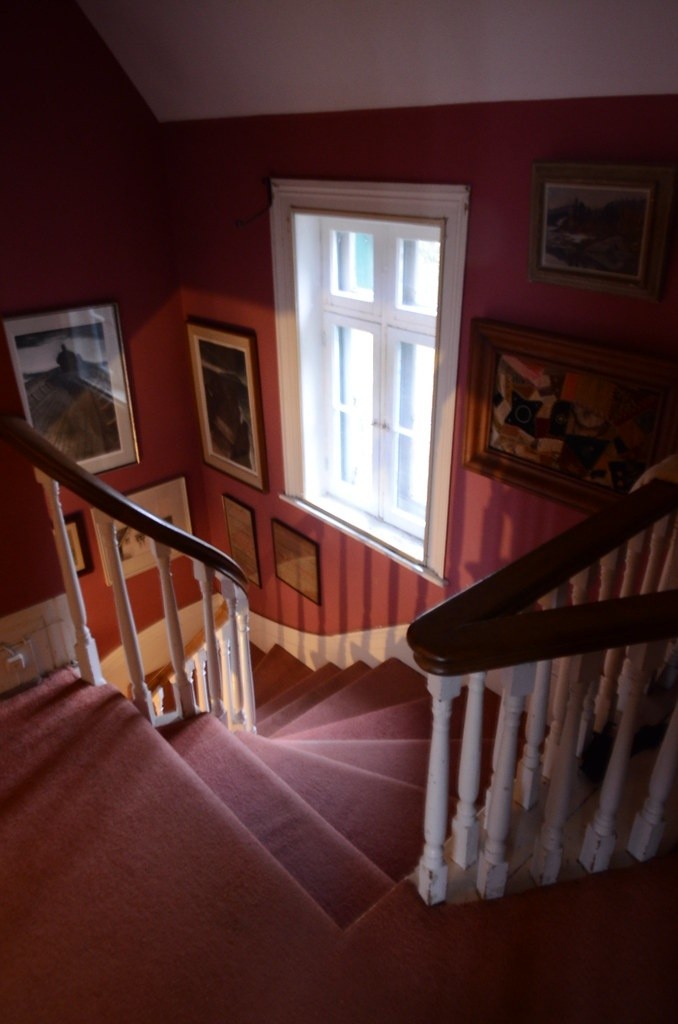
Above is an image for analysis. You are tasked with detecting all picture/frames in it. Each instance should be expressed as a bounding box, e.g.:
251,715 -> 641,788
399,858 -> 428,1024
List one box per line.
223,495 -> 262,589
185,317 -> 270,496
461,315 -> 678,519
270,516 -> 322,605
0,296 -> 140,486
524,159 -> 677,305
51,509 -> 95,578
90,477 -> 194,587
